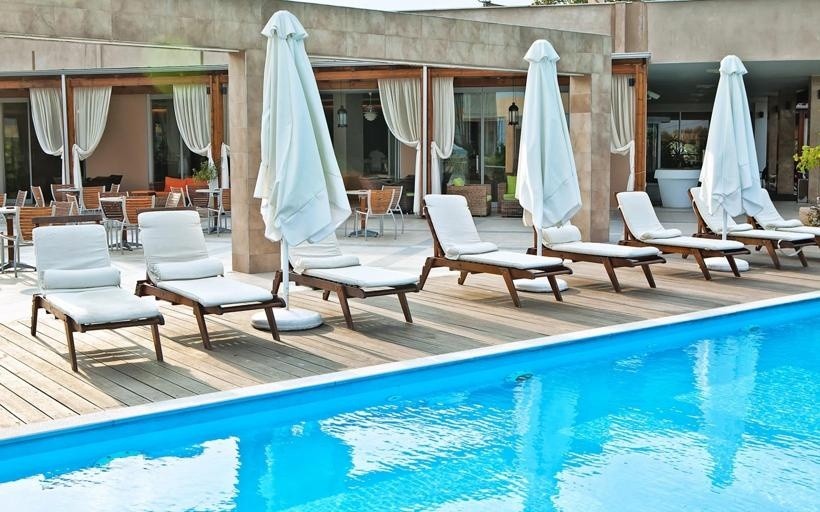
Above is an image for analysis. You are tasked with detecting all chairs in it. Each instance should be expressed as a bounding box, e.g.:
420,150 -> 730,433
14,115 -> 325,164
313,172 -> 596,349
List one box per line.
123,195 -> 157,250
355,188 -> 398,240
0,192 -> 9,208
184,184 -> 217,234
211,187 -> 231,236
99,190 -> 129,200
67,194 -> 81,218
169,185 -> 186,207
610,190 -> 750,281
50,199 -> 72,225
268,226 -> 420,331
1,205 -> 56,276
30,185 -> 46,208
135,208 -> 286,349
99,197 -> 125,251
29,225 -> 165,373
687,186 -> 816,270
165,192 -> 180,208
374,185 -> 405,234
83,186 -> 105,212
528,202 -> 667,294
413,194 -> 574,306
50,183 -> 76,202
110,183 -> 121,194
14,190 -> 28,207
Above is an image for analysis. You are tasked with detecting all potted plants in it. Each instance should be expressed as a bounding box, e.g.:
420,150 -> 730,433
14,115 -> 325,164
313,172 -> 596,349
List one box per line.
656,135 -> 702,209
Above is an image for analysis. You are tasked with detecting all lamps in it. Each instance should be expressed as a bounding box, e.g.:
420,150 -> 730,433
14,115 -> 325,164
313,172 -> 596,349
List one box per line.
335,106 -> 350,128
361,103 -> 380,122
506,102 -> 520,128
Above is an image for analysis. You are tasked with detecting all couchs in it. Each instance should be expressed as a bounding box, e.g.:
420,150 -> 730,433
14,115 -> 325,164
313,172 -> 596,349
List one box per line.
357,174 -> 419,216
446,177 -> 493,217
496,172 -> 524,218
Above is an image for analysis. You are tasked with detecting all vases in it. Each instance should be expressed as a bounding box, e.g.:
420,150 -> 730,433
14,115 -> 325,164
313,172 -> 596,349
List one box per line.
205,183 -> 217,190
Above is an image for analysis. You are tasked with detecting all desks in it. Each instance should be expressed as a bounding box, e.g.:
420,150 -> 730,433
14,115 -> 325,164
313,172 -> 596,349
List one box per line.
346,188 -> 381,238
194,186 -> 227,235
0,205 -> 28,272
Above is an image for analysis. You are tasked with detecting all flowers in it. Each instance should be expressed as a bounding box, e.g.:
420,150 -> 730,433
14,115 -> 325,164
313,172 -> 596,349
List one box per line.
192,160 -> 222,207
